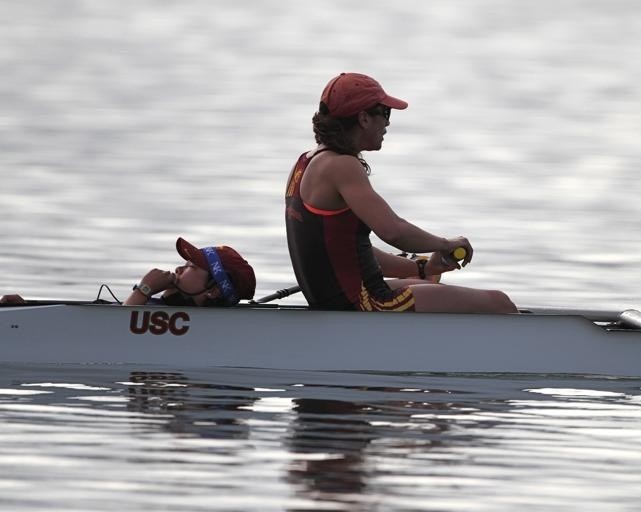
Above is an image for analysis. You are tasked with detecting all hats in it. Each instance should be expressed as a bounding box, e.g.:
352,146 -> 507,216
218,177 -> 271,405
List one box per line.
318,72 -> 408,118
176,237 -> 256,300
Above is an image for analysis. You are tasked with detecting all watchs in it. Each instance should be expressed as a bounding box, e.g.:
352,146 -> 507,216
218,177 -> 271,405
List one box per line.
133,282 -> 152,300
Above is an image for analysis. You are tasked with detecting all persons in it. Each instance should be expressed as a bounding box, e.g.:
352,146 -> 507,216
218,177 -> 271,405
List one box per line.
287,72 -> 518,310
0,237 -> 256,309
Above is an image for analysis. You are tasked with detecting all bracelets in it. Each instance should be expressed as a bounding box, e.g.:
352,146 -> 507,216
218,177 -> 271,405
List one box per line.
417,258 -> 427,279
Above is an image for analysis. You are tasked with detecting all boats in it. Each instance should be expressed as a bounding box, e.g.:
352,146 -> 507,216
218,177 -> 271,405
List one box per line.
0,290 -> 641,387
